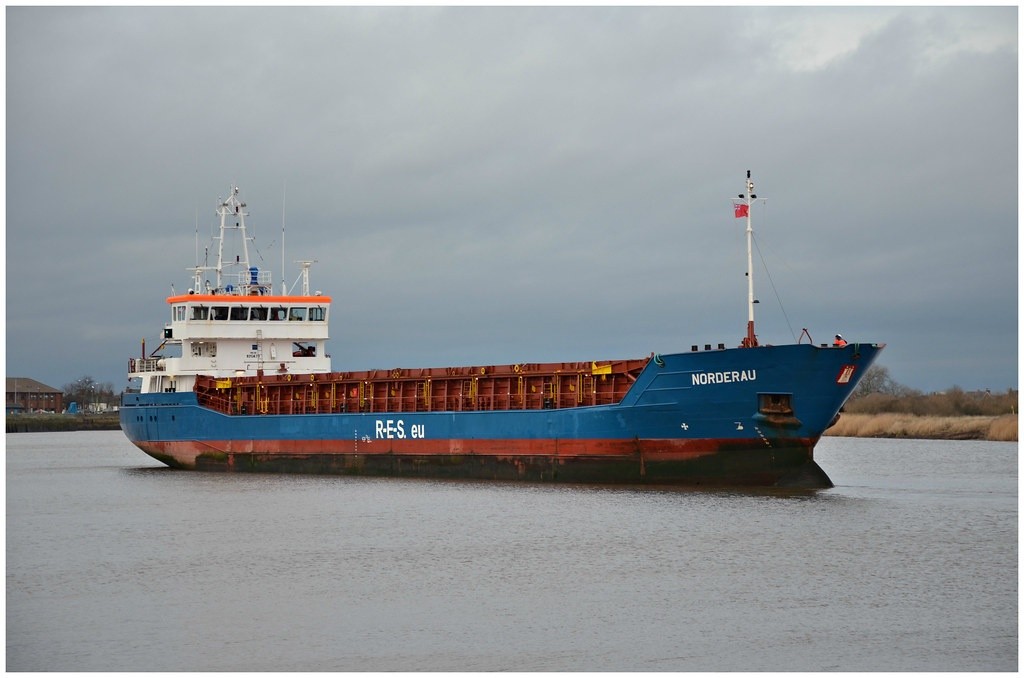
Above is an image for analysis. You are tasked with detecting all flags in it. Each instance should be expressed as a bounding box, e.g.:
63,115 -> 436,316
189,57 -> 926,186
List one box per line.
735,204 -> 749,219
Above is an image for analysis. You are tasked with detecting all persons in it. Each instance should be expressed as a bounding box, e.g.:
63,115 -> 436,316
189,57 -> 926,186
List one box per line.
834,333 -> 847,347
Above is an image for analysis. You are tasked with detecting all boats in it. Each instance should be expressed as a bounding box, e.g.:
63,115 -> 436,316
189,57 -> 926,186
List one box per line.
119,165 -> 889,491
